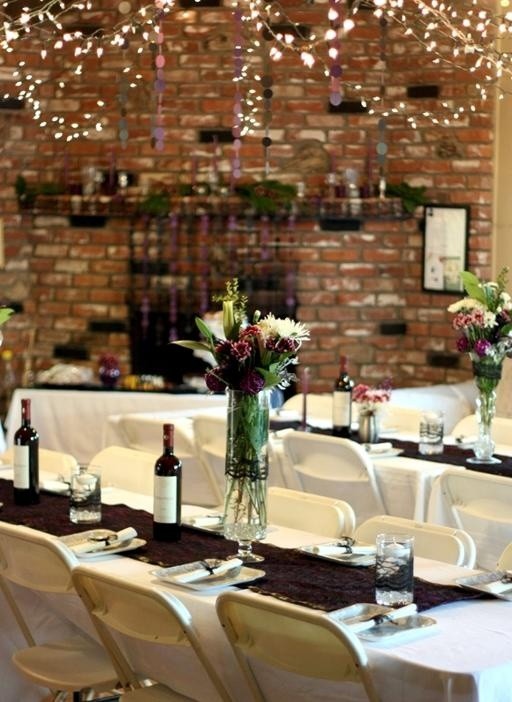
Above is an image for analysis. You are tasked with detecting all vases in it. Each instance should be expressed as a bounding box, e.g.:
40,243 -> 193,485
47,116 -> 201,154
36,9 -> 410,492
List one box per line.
349,410 -> 375,445
465,350 -> 504,469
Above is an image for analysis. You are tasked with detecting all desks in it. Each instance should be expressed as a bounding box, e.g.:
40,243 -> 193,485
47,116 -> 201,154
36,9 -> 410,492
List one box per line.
4,388 -> 228,460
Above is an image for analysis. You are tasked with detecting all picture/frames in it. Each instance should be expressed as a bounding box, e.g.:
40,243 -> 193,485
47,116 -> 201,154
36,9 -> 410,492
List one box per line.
220,388 -> 269,546
421,204 -> 468,297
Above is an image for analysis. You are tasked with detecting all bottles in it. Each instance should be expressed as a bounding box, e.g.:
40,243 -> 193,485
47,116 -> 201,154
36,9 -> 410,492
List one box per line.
153,423 -> 182,541
332,354 -> 352,436
13,398 -> 40,505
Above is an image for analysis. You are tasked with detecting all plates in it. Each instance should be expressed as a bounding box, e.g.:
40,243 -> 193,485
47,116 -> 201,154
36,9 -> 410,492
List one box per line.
323,602 -> 436,643
149,557 -> 266,592
362,447 -> 405,456
299,540 -> 377,569
60,528 -> 147,557
452,570 -> 512,603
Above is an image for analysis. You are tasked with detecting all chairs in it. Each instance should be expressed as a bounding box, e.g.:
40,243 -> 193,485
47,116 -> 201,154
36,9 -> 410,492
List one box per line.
283,431 -> 386,516
119,418 -> 218,508
189,413 -> 283,502
0,523 -> 136,702
216,582 -> 381,702
72,564 -> 235,702
283,394 -> 512,444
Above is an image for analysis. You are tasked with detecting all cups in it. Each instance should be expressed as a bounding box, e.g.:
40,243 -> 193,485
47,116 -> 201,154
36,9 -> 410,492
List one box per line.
375,533 -> 415,607
418,413 -> 444,455
68,466 -> 103,524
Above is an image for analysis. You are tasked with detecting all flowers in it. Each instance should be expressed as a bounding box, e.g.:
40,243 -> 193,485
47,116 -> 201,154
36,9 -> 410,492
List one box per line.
166,280 -> 312,529
443,264 -> 512,415
351,382 -> 391,416
